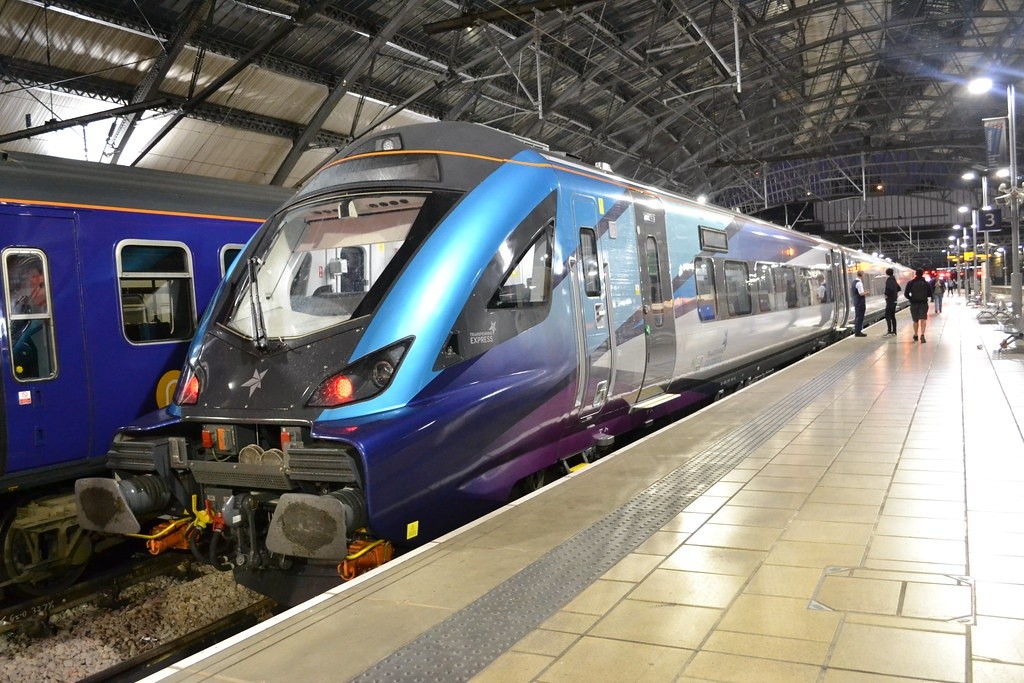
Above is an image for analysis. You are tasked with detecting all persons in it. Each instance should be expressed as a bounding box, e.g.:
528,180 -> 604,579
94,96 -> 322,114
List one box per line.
930,276 -> 935,302
905,269 -> 932,343
883,268 -> 902,337
932,274 -> 945,314
784,277 -> 799,327
851,270 -> 869,337
948,278 -> 957,296
812,275 -> 833,327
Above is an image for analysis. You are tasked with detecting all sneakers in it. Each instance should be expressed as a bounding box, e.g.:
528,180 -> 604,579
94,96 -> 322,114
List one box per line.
855,332 -> 867,337
881,332 -> 892,337
891,332 -> 897,336
913,335 -> 918,341
920,335 -> 926,343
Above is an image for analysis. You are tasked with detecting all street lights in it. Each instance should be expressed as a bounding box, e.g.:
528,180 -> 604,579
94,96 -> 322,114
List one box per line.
941,75 -> 1024,353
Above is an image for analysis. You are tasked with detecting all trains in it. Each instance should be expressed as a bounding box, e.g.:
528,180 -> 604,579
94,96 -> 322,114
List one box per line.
0,147 -> 298,601
74,119 -> 916,585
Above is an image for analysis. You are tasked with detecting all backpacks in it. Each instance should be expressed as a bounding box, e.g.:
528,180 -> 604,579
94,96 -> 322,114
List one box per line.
910,280 -> 928,302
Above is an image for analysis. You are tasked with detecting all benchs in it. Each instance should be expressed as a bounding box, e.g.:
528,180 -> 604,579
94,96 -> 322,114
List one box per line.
965,288 -> 1024,352
